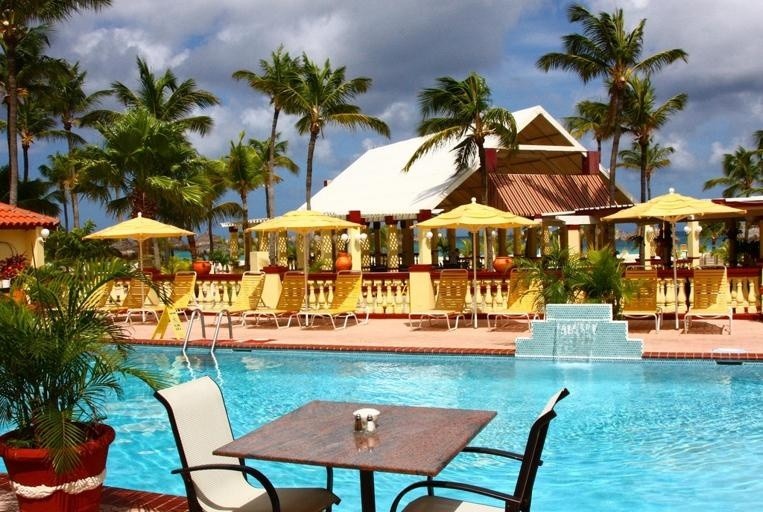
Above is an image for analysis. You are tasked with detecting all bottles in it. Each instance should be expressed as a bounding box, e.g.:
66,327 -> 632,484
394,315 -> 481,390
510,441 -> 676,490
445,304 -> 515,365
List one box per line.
366,434 -> 374,453
364,415 -> 375,432
354,434 -> 362,453
353,414 -> 362,432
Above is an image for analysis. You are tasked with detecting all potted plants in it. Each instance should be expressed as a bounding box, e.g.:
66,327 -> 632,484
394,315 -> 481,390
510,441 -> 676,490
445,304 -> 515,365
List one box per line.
0,222 -> 179,512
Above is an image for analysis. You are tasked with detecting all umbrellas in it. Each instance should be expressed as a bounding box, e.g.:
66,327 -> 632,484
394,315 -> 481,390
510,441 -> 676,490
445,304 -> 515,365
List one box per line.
80,213 -> 196,322
596,186 -> 748,330
241,205 -> 360,327
408,196 -> 536,328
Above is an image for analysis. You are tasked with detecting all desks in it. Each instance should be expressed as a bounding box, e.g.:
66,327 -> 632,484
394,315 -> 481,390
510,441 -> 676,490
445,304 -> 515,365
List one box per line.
212,400 -> 497,512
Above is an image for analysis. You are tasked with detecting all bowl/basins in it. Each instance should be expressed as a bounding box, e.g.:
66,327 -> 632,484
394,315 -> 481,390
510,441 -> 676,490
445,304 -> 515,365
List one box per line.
352,408 -> 380,422
359,435 -> 380,448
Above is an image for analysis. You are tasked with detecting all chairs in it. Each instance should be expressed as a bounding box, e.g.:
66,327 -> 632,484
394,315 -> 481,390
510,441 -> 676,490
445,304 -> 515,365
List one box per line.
487,267 -> 545,332
297,269 -> 370,330
390,388 -> 569,512
154,377 -> 341,512
195,271 -> 266,333
409,269 -> 473,330
616,265 -> 664,331
240,271 -> 308,328
684,265 -> 732,333
44,258 -> 202,331
